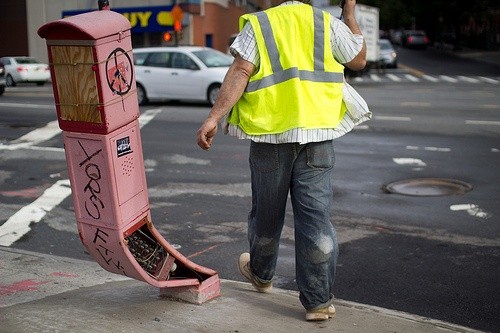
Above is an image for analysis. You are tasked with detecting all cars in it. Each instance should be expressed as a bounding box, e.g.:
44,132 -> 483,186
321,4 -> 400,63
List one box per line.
130,46 -> 241,107
0,55 -> 53,87
379,29 -> 431,49
379,39 -> 398,68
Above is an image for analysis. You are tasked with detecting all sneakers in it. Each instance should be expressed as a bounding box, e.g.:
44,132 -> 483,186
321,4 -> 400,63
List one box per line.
306,302 -> 336,321
238,251 -> 273,293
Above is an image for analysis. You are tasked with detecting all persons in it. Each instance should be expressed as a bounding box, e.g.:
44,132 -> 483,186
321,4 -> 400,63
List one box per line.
196,0 -> 373,319
